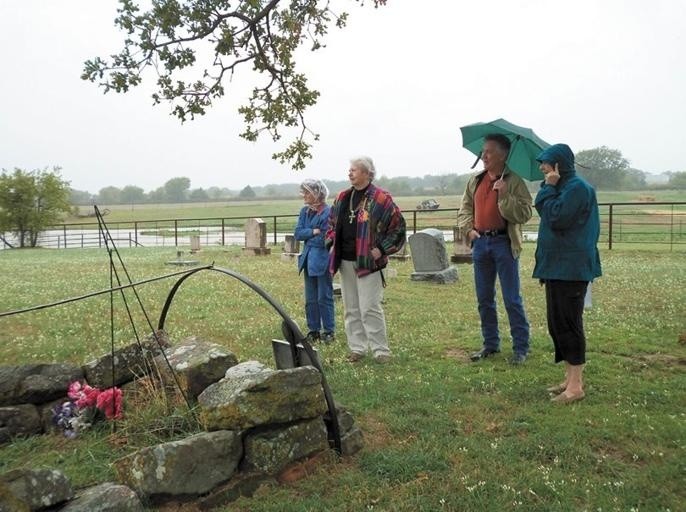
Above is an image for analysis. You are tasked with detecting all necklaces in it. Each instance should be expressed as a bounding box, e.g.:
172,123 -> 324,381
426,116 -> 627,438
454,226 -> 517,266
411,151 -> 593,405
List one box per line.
348,186 -> 371,224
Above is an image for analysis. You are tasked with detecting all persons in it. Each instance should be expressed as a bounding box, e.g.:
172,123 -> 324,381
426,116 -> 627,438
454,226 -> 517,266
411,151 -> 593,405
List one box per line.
293,177 -> 336,344
532,143 -> 603,404
325,157 -> 407,364
458,134 -> 530,365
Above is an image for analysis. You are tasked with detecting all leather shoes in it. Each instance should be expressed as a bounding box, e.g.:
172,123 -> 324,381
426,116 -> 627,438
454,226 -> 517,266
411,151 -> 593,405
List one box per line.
469,345 -> 501,362
544,382 -> 585,406
508,351 -> 528,364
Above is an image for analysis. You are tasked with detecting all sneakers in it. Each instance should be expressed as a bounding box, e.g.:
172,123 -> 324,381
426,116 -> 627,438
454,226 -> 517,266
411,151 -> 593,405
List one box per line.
343,351 -> 365,362
374,353 -> 395,364
322,331 -> 335,344
302,331 -> 320,347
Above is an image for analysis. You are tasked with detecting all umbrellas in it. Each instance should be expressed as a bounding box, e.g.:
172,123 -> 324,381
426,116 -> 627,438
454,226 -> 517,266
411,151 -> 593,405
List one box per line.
459,117 -> 552,191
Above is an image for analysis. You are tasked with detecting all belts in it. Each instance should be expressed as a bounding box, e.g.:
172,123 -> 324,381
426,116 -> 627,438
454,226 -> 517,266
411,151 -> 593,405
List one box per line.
474,228 -> 509,237
